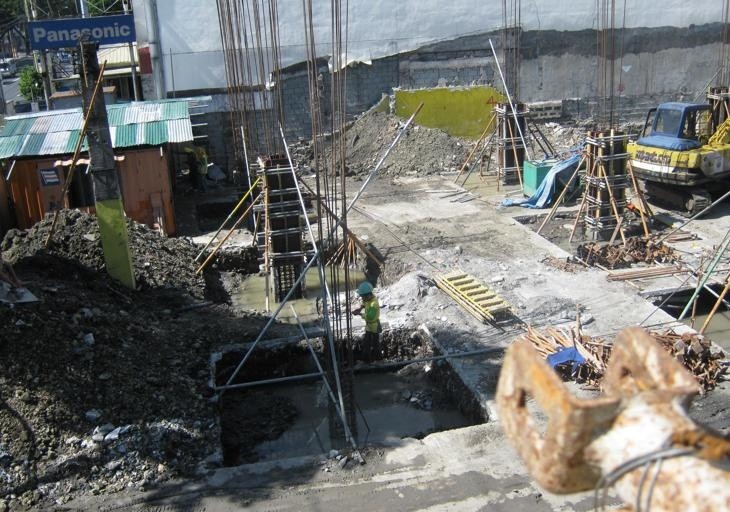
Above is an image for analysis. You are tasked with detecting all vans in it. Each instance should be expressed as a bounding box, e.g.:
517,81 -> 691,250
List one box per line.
0,58 -> 18,78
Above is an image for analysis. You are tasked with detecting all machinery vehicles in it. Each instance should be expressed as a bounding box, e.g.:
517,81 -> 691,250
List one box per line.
622,101 -> 730,218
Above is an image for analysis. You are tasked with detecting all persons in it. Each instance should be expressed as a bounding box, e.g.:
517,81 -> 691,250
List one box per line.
195,147 -> 209,196
352,281 -> 381,363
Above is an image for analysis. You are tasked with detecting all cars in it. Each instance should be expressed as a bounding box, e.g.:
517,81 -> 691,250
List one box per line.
33,48 -> 69,75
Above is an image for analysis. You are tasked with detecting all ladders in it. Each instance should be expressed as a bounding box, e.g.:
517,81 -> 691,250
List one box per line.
434,269 -> 519,324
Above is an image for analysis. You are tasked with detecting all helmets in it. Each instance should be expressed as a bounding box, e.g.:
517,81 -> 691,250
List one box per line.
357,281 -> 374,295
